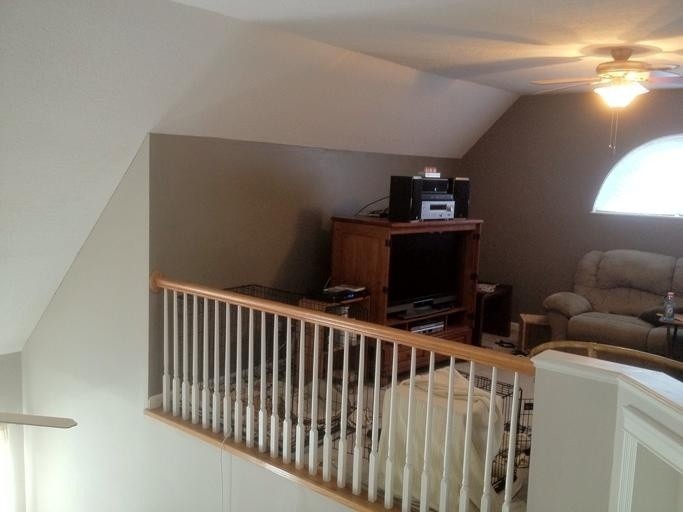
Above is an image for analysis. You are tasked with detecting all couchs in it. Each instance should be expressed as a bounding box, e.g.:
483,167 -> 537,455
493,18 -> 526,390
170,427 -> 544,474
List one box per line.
542,247 -> 682,378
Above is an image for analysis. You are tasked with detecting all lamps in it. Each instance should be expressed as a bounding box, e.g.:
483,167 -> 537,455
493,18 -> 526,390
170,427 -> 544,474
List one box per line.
592,78 -> 650,154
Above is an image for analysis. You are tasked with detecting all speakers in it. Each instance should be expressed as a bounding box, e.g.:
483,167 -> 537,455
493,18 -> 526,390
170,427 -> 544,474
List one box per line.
389,176 -> 412,222
453,178 -> 470,219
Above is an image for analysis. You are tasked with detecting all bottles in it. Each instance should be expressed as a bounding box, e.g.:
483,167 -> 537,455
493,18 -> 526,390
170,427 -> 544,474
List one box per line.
662,292 -> 675,321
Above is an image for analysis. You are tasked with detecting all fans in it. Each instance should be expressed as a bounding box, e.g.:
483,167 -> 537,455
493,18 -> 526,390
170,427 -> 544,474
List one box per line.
533,61 -> 682,95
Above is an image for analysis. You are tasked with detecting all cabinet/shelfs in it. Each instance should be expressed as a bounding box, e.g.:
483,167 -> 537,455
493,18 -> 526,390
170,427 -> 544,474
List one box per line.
297,293 -> 373,394
473,281 -> 514,347
329,213 -> 484,388
